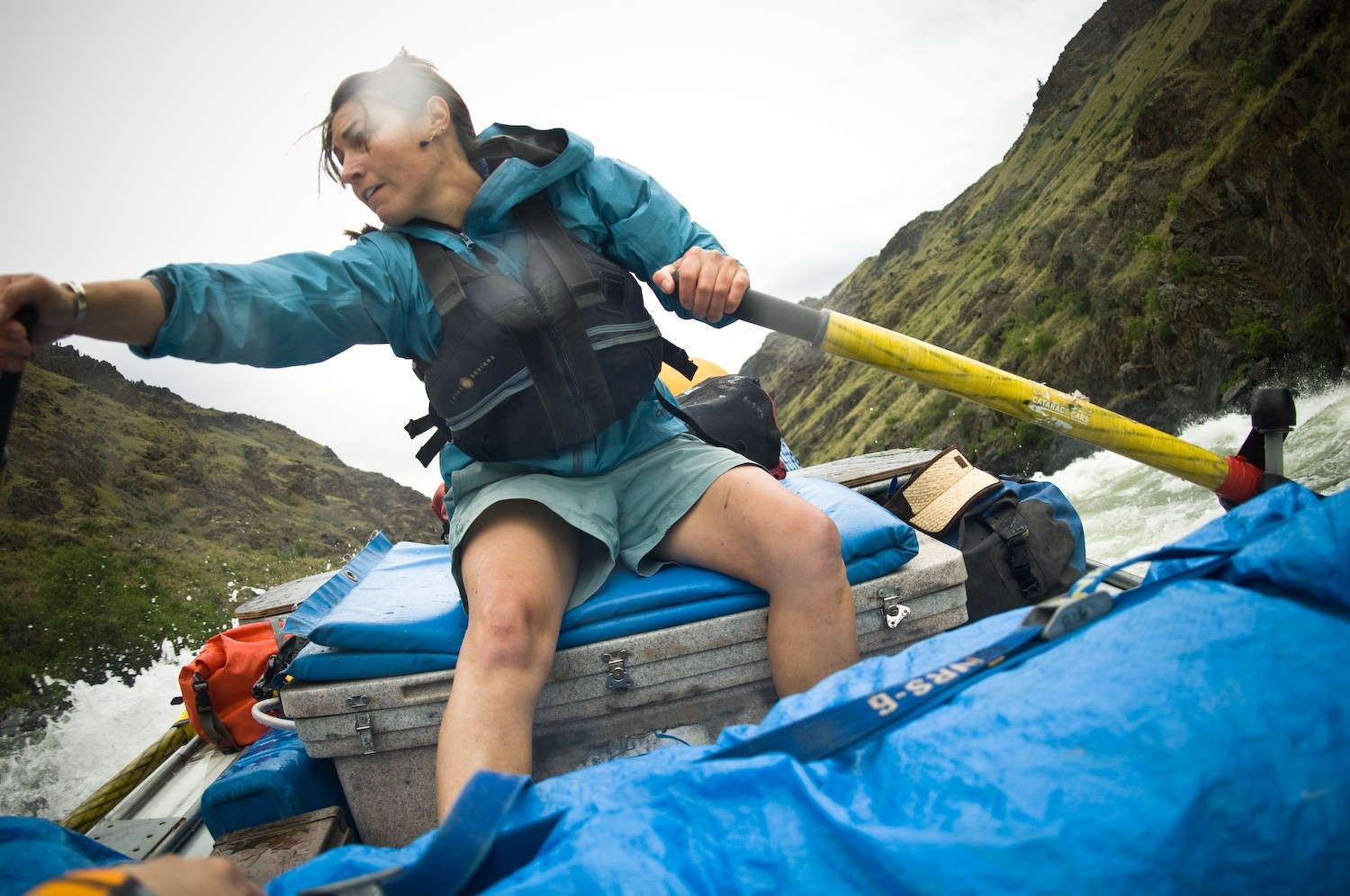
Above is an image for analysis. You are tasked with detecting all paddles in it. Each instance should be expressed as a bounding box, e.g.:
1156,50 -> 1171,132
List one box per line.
58,711 -> 196,834
0,302 -> 42,474
670,268 -> 1326,515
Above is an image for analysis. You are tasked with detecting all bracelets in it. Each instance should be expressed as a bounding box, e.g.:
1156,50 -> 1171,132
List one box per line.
62,281 -> 87,338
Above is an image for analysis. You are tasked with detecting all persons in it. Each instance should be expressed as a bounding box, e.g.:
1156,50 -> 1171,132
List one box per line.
0,51 -> 860,827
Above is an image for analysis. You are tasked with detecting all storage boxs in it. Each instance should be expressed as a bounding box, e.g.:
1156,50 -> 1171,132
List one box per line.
200,714 -> 361,844
278,523 -> 974,846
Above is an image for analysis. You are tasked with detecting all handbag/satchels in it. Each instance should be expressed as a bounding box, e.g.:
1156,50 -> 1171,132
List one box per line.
942,472 -> 1086,620
178,623 -> 286,755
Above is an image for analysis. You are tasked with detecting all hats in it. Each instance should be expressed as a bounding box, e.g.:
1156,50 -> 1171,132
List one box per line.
886,442 -> 1003,537
